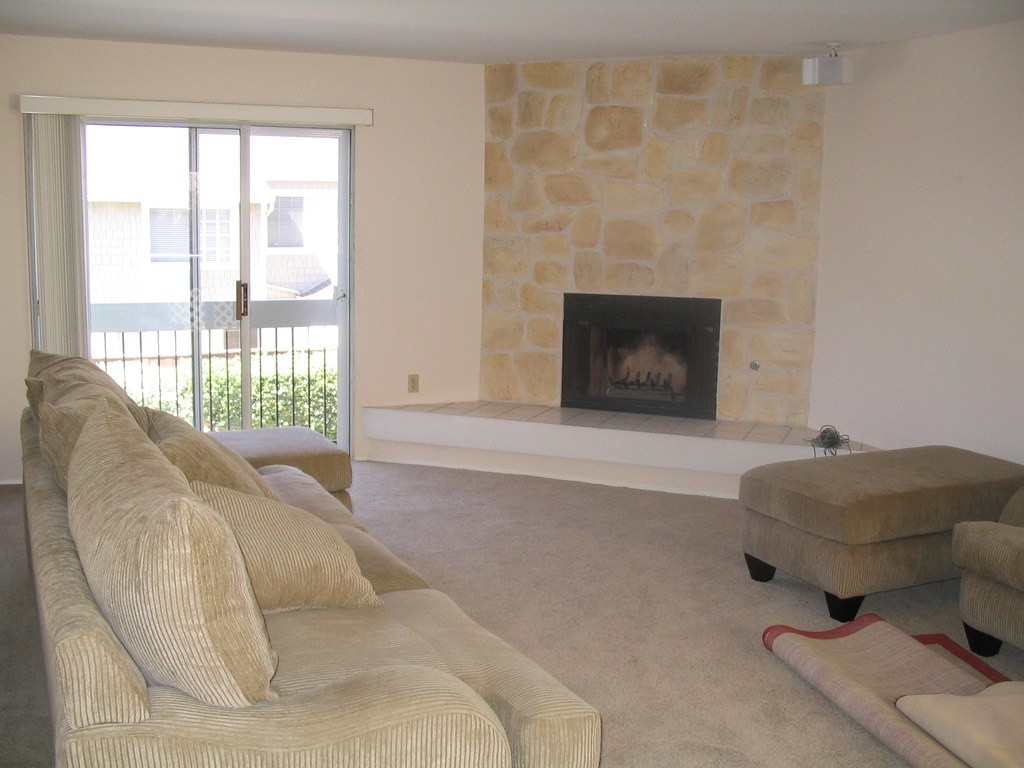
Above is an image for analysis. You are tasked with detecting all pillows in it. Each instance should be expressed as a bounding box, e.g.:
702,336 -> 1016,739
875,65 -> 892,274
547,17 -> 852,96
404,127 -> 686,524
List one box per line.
23,347 -> 384,708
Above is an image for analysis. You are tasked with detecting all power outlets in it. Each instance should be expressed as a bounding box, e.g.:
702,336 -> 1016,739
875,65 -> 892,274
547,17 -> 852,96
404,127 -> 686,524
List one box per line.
407,374 -> 419,394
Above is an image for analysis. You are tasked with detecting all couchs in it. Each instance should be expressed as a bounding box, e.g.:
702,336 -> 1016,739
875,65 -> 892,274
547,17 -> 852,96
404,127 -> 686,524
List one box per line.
952,487 -> 1024,657
16,347 -> 602,768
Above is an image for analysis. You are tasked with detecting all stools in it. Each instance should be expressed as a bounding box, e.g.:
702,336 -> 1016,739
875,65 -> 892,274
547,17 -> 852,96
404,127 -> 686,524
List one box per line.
205,425 -> 353,516
739,445 -> 1024,623
